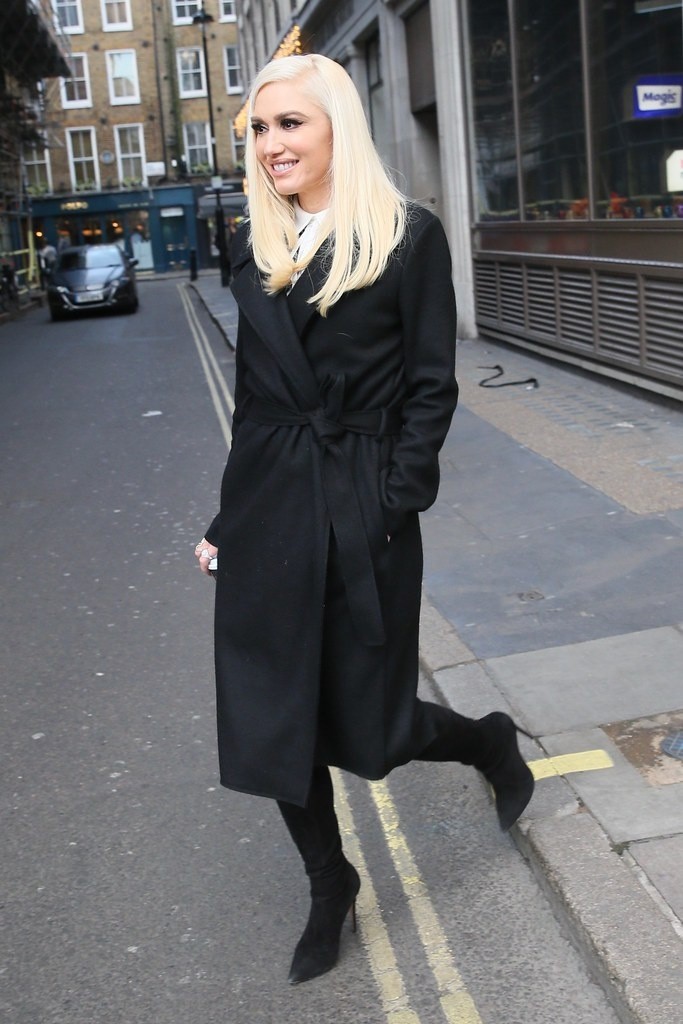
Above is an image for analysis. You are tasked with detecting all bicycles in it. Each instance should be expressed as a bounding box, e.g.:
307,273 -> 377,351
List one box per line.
0,264 -> 25,322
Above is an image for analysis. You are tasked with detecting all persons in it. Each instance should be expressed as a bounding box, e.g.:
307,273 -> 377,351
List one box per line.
131,228 -> 144,244
0,255 -> 23,293
40,236 -> 70,284
195,53 -> 535,985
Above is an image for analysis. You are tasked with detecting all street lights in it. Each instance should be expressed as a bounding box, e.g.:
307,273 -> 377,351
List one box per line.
191,5 -> 233,289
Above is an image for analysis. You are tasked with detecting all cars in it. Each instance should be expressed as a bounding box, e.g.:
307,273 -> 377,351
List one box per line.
48,244 -> 142,321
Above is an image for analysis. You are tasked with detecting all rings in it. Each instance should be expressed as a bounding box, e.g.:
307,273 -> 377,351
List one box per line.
201,549 -> 214,560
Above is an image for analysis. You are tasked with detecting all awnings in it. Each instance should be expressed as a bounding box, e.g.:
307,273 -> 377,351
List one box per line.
196,193 -> 248,218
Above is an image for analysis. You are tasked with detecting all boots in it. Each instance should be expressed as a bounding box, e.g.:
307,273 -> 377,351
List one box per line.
411,702 -> 535,833
276,767 -> 360,985
